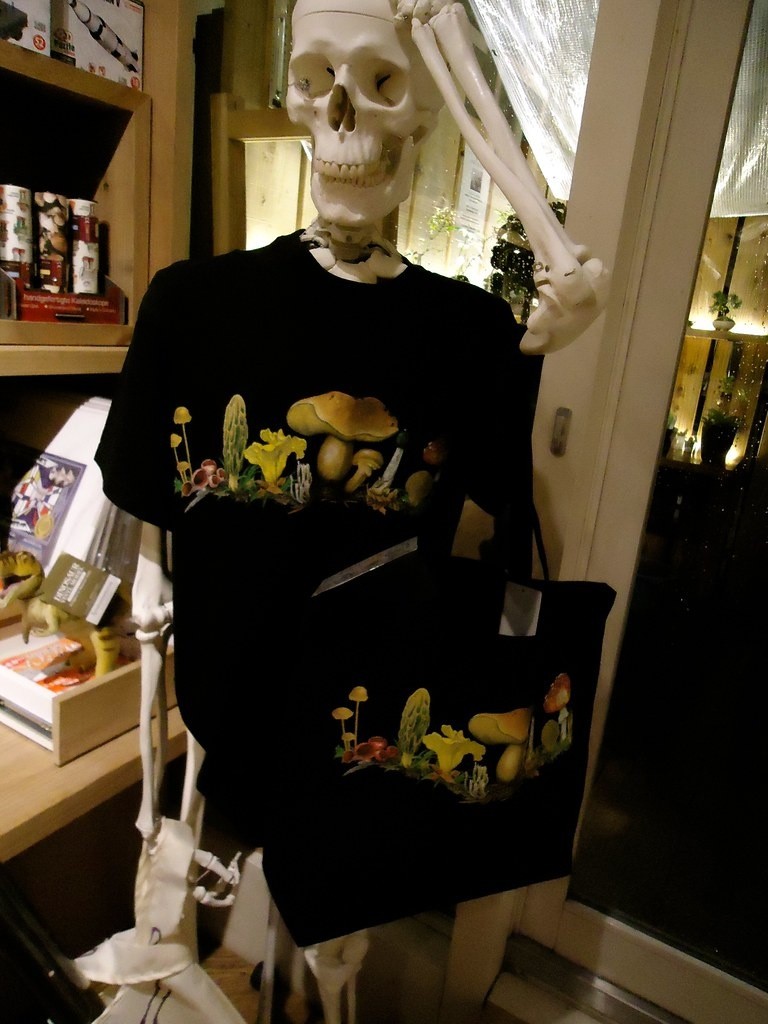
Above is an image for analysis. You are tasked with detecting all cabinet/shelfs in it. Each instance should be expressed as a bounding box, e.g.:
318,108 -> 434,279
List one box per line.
0,1 -> 224,865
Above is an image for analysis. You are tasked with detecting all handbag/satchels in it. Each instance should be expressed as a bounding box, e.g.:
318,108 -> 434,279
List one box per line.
261,491 -> 616,950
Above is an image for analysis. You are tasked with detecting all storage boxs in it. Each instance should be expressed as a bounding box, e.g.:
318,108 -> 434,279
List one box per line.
15,274 -> 124,323
0,1 -> 174,93
